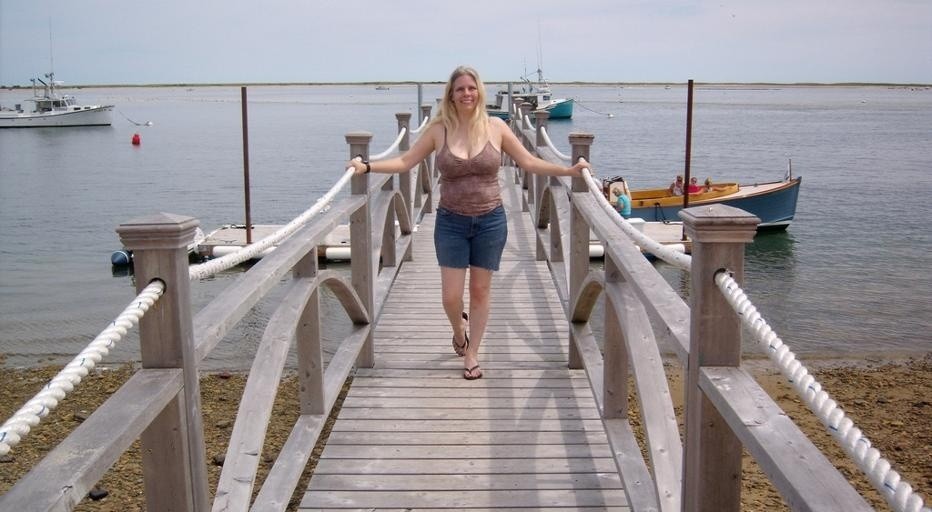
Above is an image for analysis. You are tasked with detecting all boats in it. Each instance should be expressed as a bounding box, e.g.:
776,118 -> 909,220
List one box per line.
485,65 -> 573,119
0,73 -> 113,126
592,159 -> 802,230
376,85 -> 391,90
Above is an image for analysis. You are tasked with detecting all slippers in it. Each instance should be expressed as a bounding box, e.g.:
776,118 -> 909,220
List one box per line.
452,312 -> 469,356
464,364 -> 482,380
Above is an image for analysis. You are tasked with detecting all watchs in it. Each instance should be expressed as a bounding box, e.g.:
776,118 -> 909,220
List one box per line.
363,160 -> 370,174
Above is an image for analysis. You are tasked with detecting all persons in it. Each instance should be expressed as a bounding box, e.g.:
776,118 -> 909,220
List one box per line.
345,66 -> 592,381
665,175 -> 727,197
613,187 -> 631,219
